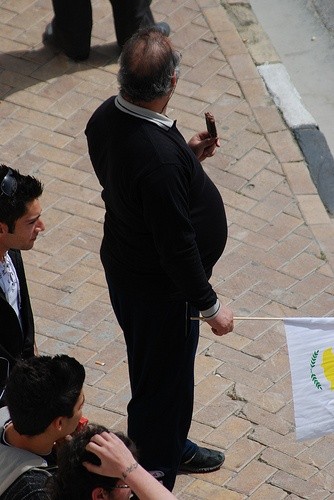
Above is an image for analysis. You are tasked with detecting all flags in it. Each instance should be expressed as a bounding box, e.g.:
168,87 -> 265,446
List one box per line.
283,317 -> 334,440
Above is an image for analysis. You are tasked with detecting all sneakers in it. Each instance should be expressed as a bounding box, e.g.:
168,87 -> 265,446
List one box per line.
177,443 -> 225,472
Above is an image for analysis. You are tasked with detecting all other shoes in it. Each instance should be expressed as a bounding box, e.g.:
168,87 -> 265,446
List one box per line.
156,22 -> 170,37
46,23 -> 88,61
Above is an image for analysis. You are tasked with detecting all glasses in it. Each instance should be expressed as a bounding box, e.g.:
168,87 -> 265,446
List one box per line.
0,167 -> 17,197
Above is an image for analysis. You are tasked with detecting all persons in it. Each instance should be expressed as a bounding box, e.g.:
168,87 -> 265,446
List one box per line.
43,0 -> 170,62
84,28 -> 233,475
0,165 -> 184,500
